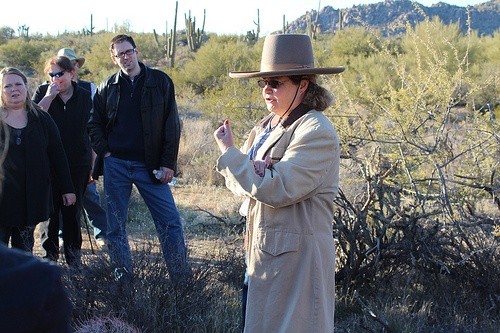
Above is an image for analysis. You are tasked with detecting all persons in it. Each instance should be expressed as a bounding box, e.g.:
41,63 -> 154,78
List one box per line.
213,35 -> 346,333
42,48 -> 110,260
30,56 -> 92,271
0,68 -> 76,254
86,35 -> 193,283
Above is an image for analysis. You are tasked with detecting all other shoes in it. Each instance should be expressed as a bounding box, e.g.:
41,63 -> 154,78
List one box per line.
96,238 -> 108,252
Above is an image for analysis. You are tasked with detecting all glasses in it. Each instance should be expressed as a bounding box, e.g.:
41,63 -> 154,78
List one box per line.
114,49 -> 135,56
48,69 -> 67,78
257,77 -> 291,88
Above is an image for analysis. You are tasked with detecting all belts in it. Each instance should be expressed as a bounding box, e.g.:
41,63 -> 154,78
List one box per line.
112,152 -> 146,161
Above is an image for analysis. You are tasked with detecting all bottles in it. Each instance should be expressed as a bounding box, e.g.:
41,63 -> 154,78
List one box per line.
153,170 -> 178,187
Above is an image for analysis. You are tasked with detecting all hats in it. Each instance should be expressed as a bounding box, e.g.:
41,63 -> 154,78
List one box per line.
57,47 -> 85,68
228,34 -> 346,79
